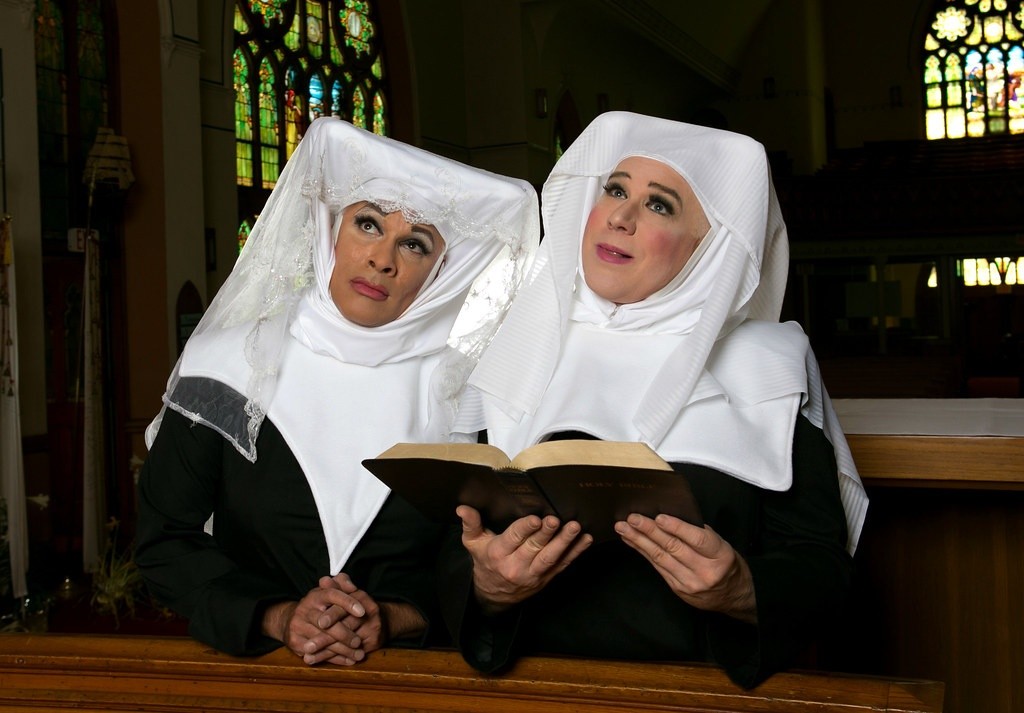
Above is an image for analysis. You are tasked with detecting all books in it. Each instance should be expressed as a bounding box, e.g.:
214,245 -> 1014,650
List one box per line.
360,440 -> 703,547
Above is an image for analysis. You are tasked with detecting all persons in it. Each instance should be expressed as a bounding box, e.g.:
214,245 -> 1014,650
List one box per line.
423,111 -> 900,689
128,116 -> 541,665
